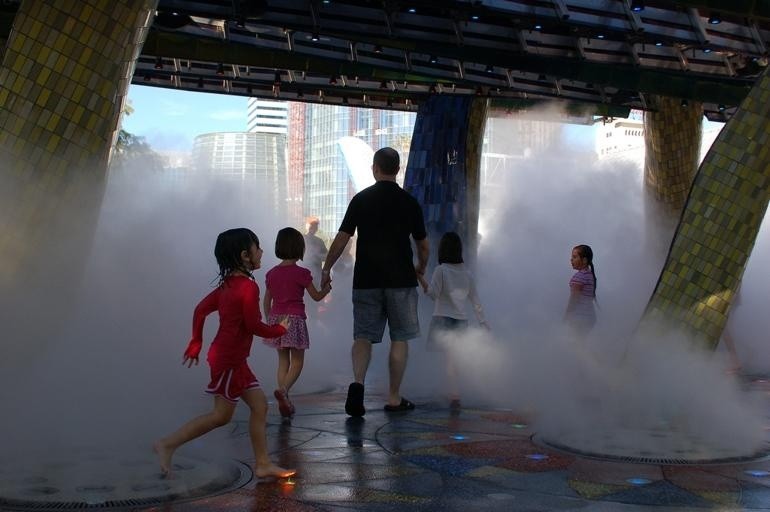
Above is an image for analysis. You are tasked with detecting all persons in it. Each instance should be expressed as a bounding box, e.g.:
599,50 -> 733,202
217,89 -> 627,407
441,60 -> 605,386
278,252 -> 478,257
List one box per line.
321,147 -> 429,415
153,227 -> 299,478
417,232 -> 493,411
303,218 -> 330,318
264,226 -> 331,418
559,244 -> 598,380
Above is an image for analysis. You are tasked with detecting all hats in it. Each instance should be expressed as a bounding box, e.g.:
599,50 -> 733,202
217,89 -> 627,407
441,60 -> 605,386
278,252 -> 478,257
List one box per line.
307,217 -> 319,225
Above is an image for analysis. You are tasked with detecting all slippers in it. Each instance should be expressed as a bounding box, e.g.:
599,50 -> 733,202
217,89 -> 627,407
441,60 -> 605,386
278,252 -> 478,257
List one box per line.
346,383 -> 365,417
385,398 -> 415,412
274,389 -> 292,417
